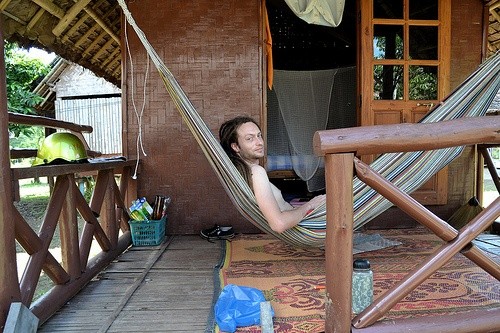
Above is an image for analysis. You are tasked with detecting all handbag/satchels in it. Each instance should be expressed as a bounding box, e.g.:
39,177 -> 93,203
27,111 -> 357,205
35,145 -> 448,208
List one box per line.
215,283 -> 275,333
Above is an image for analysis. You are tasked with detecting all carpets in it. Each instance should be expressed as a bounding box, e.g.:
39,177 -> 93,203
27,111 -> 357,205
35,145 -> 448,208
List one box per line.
205,234 -> 500,333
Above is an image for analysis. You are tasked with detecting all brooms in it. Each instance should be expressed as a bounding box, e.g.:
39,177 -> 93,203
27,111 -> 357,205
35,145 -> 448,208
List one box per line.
446,142 -> 486,228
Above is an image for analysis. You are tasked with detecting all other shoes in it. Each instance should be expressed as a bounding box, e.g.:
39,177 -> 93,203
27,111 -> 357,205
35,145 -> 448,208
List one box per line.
201,225 -> 235,243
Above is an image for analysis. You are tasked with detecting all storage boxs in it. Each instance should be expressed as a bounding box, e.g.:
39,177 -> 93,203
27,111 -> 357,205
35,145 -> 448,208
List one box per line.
128,214 -> 167,247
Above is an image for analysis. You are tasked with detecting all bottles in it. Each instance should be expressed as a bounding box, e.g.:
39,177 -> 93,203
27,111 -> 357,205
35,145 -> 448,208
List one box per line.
130,210 -> 146,220
153,196 -> 165,220
352,257 -> 374,314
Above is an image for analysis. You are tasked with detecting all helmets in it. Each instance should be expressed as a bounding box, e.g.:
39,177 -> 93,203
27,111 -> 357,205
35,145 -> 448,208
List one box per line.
31,132 -> 89,167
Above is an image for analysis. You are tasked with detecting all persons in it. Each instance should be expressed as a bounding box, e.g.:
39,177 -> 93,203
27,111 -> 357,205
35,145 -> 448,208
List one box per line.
219,101 -> 445,233
